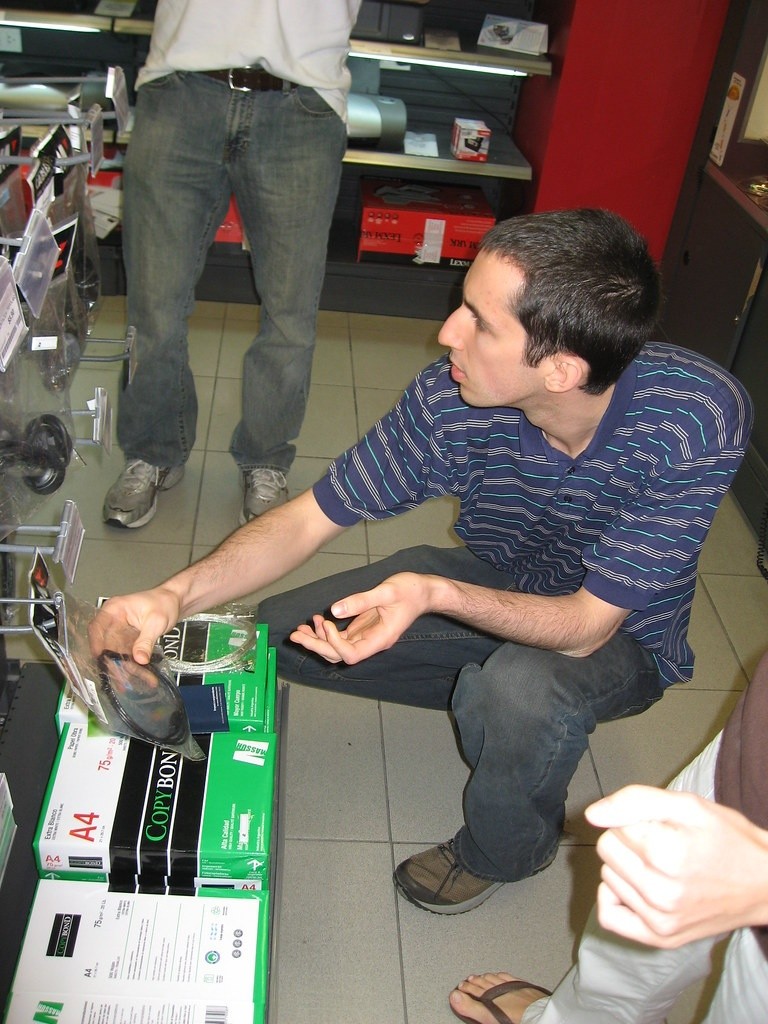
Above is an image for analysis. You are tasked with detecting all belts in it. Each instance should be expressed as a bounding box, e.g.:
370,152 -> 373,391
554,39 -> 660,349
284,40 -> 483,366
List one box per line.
196,66 -> 299,93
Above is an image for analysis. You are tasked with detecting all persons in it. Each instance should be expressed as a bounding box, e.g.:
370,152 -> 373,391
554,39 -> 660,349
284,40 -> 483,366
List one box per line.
450,649 -> 768,1024
89,209 -> 752,913
103,0 -> 365,530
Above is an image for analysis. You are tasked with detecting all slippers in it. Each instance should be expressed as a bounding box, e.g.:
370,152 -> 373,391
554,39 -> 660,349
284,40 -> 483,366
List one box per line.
449,971 -> 554,1024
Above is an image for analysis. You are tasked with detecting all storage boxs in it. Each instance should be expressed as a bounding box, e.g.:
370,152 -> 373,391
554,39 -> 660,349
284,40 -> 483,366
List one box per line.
359,176 -> 495,270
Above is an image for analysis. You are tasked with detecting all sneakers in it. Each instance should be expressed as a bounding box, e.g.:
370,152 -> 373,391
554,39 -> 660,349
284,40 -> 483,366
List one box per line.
392,839 -> 563,915
239,467 -> 290,525
102,458 -> 184,530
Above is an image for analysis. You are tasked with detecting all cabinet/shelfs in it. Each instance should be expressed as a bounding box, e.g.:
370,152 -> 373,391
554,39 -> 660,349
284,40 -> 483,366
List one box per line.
0,1 -> 558,319
650,161 -> 767,552
